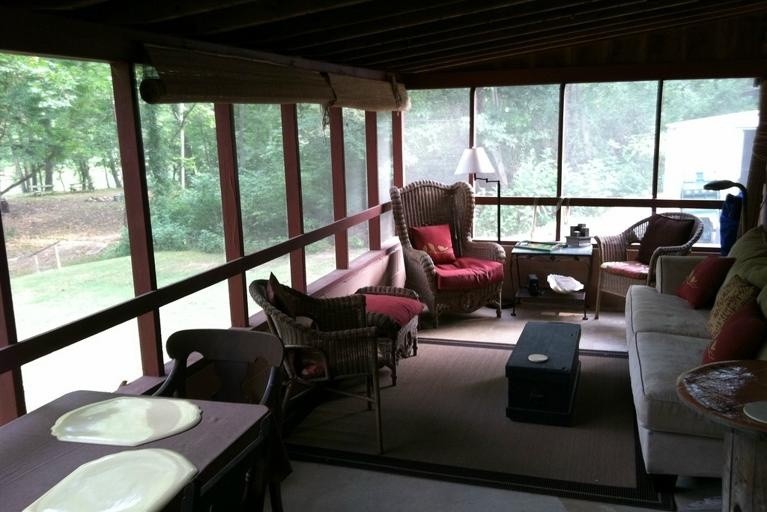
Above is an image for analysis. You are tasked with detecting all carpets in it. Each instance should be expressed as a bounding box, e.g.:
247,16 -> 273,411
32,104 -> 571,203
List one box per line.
271,337 -> 678,511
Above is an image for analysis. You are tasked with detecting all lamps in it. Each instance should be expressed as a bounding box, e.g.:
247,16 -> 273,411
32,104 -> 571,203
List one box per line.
704,180 -> 748,235
455,147 -> 502,243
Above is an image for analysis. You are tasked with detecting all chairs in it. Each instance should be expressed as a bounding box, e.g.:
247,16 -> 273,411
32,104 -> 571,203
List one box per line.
249,279 -> 385,455
150,329 -> 286,510
594,213 -> 704,319
390,181 -> 507,329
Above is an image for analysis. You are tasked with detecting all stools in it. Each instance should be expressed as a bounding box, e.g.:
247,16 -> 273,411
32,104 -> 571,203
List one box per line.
354,285 -> 420,386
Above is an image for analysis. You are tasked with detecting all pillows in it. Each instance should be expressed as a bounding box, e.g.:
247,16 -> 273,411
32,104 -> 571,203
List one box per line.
702,298 -> 767,364
678,257 -> 735,310
266,272 -> 295,320
409,224 -> 456,265
705,274 -> 761,340
356,294 -> 429,332
638,215 -> 693,265
720,192 -> 744,256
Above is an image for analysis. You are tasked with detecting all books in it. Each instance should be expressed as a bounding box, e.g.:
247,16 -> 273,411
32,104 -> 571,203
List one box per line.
515,240 -> 563,253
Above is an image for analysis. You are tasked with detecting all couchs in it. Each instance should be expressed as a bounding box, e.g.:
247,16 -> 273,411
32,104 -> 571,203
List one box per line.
625,225 -> 767,487
